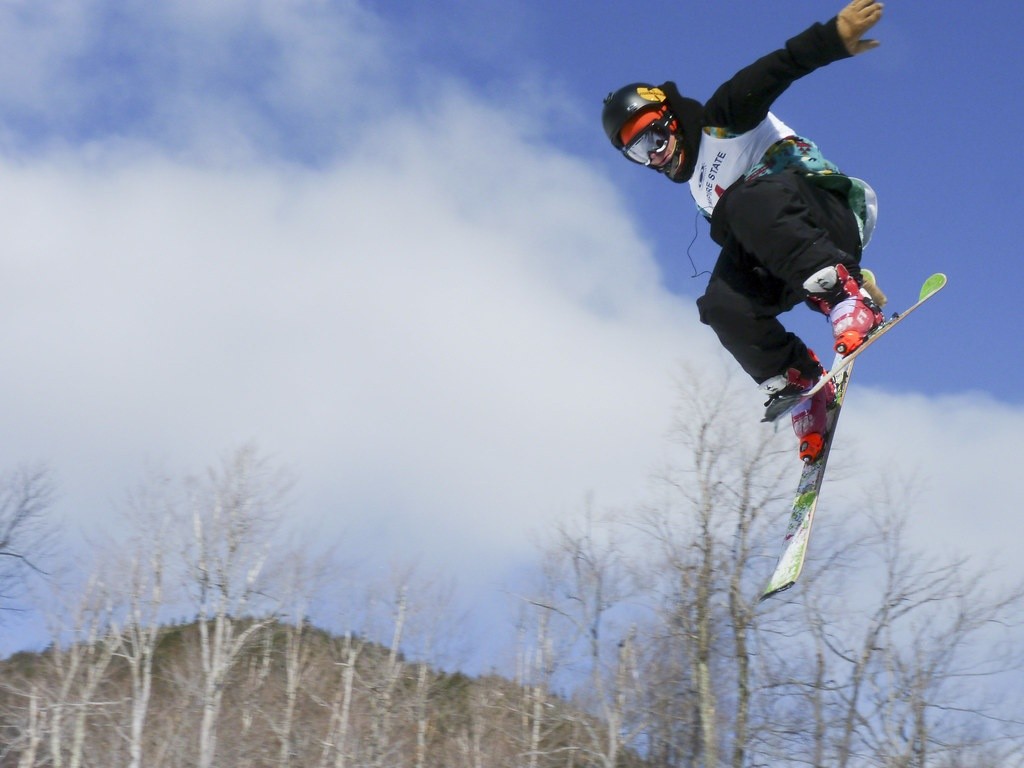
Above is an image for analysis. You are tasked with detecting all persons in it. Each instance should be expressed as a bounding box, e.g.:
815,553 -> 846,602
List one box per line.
600,0 -> 886,467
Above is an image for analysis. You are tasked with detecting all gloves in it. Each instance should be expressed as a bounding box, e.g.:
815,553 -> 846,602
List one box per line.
837,0 -> 883,57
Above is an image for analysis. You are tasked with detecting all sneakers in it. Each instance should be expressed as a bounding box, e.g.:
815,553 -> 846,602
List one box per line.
781,348 -> 835,438
801,264 -> 882,337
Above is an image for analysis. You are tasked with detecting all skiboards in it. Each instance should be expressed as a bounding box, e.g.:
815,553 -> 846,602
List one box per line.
758,268 -> 947,603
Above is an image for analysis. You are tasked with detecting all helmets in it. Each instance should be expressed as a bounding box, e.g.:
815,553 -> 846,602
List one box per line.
602,83 -> 666,150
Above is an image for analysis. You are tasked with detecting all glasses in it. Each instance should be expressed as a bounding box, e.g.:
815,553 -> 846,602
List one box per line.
621,119 -> 670,167
